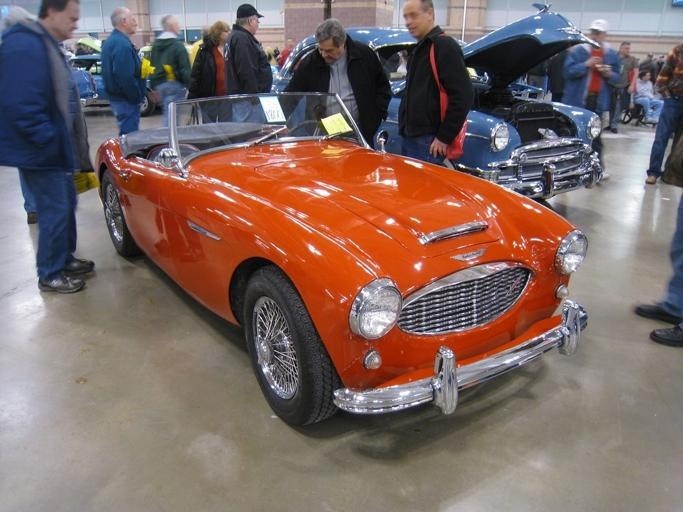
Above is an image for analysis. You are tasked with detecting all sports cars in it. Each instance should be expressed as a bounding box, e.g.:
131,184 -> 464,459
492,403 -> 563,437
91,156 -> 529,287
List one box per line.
95,91 -> 589,429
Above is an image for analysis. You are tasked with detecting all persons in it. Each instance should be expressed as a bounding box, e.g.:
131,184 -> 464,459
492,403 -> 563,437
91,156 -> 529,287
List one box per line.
99,7 -> 156,137
397,0 -> 474,168
3,4 -> 59,226
188,21 -> 233,124
277,19 -> 391,153
62,34 -> 101,73
260,35 -> 295,78
1,1 -> 95,297
222,3 -> 272,127
149,14 -> 190,128
645,43 -> 683,186
525,18 -> 683,135
630,190 -> 683,347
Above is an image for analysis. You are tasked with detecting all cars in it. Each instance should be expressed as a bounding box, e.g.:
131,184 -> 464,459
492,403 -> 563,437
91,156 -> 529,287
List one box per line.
62,53 -> 153,116
272,4 -> 601,203
138,42 -> 192,78
69,36 -> 103,73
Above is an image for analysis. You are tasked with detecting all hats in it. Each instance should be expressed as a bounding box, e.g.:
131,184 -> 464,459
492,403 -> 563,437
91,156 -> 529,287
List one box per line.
237,4 -> 264,19
590,20 -> 607,32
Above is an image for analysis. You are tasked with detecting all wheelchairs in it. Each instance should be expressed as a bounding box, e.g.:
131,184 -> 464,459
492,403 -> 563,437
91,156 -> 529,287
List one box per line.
620,101 -> 655,127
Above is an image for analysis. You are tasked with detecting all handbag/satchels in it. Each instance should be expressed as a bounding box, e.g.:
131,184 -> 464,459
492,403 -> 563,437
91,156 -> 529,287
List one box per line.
440,89 -> 468,159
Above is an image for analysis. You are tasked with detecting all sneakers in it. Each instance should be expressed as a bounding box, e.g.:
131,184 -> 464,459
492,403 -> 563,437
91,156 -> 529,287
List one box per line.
28,213 -> 38,224
646,176 -> 656,184
635,302 -> 680,324
63,257 -> 94,274
38,274 -> 83,293
650,323 -> 682,347
